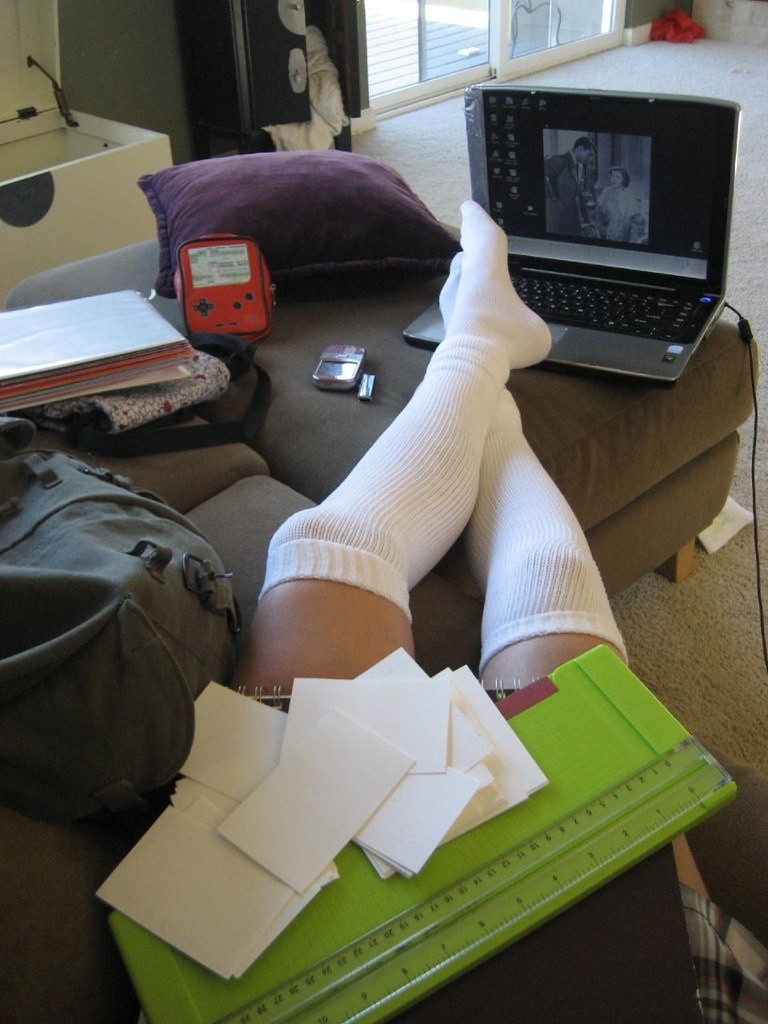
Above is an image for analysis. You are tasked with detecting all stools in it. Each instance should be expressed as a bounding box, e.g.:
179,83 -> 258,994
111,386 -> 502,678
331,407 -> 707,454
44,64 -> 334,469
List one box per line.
28,226 -> 758,675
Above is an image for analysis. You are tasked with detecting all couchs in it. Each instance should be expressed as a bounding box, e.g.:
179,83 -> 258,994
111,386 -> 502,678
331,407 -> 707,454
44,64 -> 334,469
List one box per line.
0,309 -> 768,1024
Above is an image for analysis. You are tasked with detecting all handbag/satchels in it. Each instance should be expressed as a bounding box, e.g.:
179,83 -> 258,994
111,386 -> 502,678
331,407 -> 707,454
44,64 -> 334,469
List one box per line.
0,417 -> 243,827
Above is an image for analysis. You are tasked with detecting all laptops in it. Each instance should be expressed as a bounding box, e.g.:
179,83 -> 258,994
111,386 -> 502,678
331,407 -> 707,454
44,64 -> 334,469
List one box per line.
404,83 -> 741,386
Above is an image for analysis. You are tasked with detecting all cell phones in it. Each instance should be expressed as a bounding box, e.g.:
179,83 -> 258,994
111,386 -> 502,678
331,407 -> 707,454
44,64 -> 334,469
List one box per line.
312,344 -> 366,391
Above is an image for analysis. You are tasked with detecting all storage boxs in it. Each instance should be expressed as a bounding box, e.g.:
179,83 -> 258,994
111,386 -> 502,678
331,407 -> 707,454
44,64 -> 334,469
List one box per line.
0,0 -> 174,311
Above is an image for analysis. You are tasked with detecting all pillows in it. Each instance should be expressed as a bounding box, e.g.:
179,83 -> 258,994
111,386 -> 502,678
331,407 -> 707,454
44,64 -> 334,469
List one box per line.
136,150 -> 462,299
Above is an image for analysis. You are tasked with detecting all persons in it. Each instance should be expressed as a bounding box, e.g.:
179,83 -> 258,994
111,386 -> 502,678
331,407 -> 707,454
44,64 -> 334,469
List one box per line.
230,202 -> 768,1024
544,136 -> 596,237
592,166 -> 642,242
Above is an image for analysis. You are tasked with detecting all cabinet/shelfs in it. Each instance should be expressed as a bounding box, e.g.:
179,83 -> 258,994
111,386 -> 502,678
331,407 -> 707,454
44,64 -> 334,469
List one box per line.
176,0 -> 369,155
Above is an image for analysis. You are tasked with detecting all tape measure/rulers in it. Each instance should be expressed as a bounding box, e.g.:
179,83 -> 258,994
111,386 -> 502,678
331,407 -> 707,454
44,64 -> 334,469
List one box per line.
213,736 -> 734,1024
